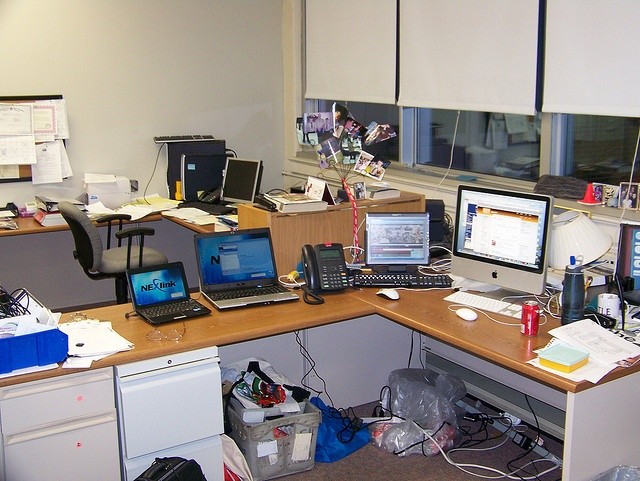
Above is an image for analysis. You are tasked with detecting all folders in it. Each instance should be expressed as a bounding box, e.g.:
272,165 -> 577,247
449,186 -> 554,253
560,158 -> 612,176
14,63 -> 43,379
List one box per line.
34,195 -> 87,212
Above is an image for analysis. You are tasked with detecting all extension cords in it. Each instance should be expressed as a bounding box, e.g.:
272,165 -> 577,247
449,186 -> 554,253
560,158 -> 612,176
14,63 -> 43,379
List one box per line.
360,417 -> 402,424
502,412 -> 521,427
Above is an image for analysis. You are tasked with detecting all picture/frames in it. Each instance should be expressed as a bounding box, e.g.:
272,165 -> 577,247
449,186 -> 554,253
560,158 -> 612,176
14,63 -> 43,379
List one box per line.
619,182 -> 639,210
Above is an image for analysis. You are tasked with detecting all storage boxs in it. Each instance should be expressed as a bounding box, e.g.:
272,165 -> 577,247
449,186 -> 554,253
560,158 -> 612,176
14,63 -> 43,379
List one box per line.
224,400 -> 322,481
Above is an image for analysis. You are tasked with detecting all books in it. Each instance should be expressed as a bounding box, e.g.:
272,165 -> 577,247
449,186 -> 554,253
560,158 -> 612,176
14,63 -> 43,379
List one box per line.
31,208 -> 70,228
367,183 -> 401,200
263,192 -> 328,215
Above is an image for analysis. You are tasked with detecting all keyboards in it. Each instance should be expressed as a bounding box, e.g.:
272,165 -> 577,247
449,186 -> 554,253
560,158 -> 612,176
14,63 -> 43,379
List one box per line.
177,200 -> 234,215
442,292 -> 522,321
154,135 -> 214,144
353,274 -> 451,288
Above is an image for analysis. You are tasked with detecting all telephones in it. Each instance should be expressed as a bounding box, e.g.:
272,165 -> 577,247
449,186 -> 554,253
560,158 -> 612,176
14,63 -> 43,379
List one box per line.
300,243 -> 349,305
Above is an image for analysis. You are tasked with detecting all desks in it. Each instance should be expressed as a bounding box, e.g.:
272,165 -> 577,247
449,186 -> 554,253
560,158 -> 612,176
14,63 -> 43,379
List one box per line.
345,257 -> 639,481
162,210 -> 215,234
0,279 -> 377,387
1,214 -> 162,236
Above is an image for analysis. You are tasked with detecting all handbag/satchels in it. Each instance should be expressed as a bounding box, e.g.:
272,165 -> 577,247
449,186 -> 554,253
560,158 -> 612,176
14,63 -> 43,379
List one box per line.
132,457 -> 206,480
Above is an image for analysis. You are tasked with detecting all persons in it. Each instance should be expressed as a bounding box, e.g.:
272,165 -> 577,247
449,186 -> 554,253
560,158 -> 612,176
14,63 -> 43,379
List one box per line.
343,119 -> 364,140
306,118 -> 317,134
323,118 -> 333,134
369,161 -> 384,176
316,114 -> 325,136
621,184 -> 637,207
301,133 -> 309,143
594,187 -> 602,201
334,105 -> 347,136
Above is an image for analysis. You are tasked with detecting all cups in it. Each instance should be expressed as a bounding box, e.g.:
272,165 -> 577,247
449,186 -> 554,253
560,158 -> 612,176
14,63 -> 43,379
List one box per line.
561,264 -> 587,324
597,291 -> 630,321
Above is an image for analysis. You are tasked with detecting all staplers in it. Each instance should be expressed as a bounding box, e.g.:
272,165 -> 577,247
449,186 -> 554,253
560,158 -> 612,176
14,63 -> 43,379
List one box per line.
252,196 -> 278,213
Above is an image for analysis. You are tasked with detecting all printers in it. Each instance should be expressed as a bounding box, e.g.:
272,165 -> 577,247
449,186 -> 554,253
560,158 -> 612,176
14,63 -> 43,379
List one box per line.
78,173 -> 133,215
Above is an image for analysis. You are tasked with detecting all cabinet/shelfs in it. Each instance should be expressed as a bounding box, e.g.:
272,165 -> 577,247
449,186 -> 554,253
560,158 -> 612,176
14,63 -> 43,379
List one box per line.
237,190 -> 427,275
114,345 -> 224,481
1,367 -> 121,480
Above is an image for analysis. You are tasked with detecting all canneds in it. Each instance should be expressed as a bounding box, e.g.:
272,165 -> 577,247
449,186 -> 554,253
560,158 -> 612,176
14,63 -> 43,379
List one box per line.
520,300 -> 539,337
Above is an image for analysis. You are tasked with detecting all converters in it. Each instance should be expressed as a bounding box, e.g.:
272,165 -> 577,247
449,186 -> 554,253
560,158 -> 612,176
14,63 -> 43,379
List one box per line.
349,418 -> 363,431
426,200 -> 445,221
512,432 -> 532,450
462,411 -> 479,423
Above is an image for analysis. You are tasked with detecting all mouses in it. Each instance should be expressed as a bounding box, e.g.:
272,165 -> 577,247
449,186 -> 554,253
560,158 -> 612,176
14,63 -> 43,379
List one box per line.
455,308 -> 478,322
375,289 -> 400,301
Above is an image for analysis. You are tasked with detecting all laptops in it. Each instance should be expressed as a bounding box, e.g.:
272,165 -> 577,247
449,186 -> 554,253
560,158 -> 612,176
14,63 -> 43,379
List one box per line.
125,260 -> 212,326
193,226 -> 300,312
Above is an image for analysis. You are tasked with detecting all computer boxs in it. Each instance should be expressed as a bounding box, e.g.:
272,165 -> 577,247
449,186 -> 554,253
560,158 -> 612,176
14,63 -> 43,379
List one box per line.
180,153 -> 233,201
167,141 -> 225,200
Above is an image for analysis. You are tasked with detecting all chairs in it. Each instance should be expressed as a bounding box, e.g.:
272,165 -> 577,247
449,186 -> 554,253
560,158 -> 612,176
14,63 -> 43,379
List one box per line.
57,202 -> 168,303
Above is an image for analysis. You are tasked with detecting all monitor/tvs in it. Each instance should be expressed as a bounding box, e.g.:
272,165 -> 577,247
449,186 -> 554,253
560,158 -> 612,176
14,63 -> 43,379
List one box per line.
220,158 -> 264,204
363,211 -> 431,275
451,184 -> 555,304
